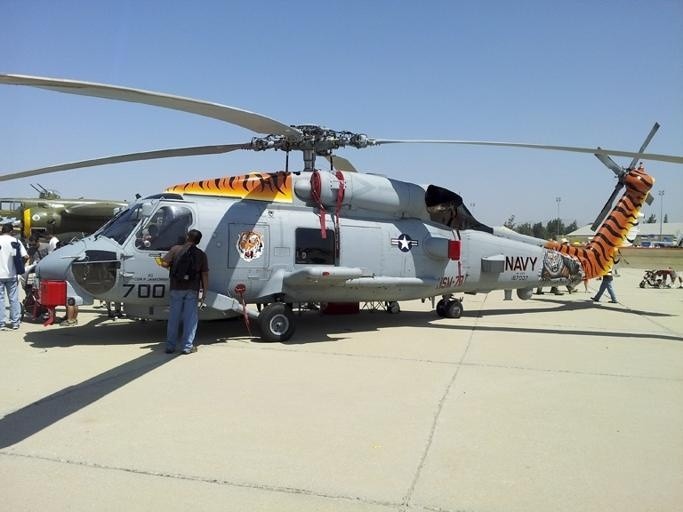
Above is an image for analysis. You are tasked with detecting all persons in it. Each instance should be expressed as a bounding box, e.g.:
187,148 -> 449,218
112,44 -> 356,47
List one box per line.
45,230 -> 59,256
60,305 -> 78,326
535,269 -> 617,303
26,234 -> 53,266
0,223 -> 29,331
159,228 -> 210,354
147,224 -> 157,243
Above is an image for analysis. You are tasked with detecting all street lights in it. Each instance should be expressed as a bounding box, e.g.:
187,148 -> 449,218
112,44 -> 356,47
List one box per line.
555,195 -> 561,235
657,189 -> 664,242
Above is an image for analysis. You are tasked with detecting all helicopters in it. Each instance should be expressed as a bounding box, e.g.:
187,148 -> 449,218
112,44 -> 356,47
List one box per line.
0,73 -> 683,342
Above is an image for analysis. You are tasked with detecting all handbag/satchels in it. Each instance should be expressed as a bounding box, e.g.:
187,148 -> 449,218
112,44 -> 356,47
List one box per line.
12,255 -> 26,275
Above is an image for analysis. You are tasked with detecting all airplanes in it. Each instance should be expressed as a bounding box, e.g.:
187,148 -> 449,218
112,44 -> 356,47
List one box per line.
0,183 -> 130,243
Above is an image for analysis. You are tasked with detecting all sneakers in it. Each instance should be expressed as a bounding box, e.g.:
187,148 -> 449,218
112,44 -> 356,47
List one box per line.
166,347 -> 196,354
31,317 -> 78,327
537,288 -> 593,295
590,297 -> 619,303
0,322 -> 20,330
